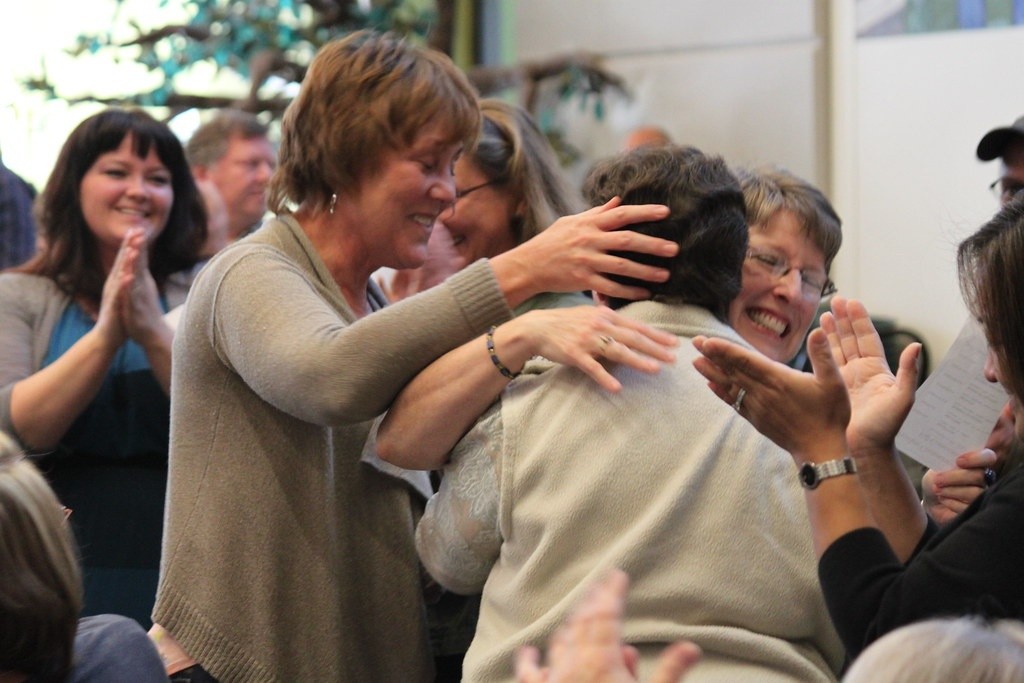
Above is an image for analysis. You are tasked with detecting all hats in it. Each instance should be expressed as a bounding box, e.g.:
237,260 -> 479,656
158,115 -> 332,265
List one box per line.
978,117 -> 1024,161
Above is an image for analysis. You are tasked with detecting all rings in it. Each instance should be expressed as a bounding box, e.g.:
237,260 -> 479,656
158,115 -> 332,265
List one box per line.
985,468 -> 997,481
599,334 -> 615,356
732,387 -> 745,412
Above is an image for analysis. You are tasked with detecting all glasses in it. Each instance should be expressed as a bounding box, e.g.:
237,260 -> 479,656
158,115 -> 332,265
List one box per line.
436,174 -> 504,223
744,250 -> 836,300
990,180 -> 1024,200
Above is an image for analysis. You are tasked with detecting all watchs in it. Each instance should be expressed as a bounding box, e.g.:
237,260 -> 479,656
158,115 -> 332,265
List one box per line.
798,456 -> 858,490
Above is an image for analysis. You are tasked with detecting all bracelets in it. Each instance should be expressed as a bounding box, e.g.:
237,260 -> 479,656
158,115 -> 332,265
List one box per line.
486,324 -> 520,379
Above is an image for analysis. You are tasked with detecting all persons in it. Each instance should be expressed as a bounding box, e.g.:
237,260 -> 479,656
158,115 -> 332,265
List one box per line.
687,103 -> 1024,683
150,27 -> 843,683
0,97 -> 276,683
